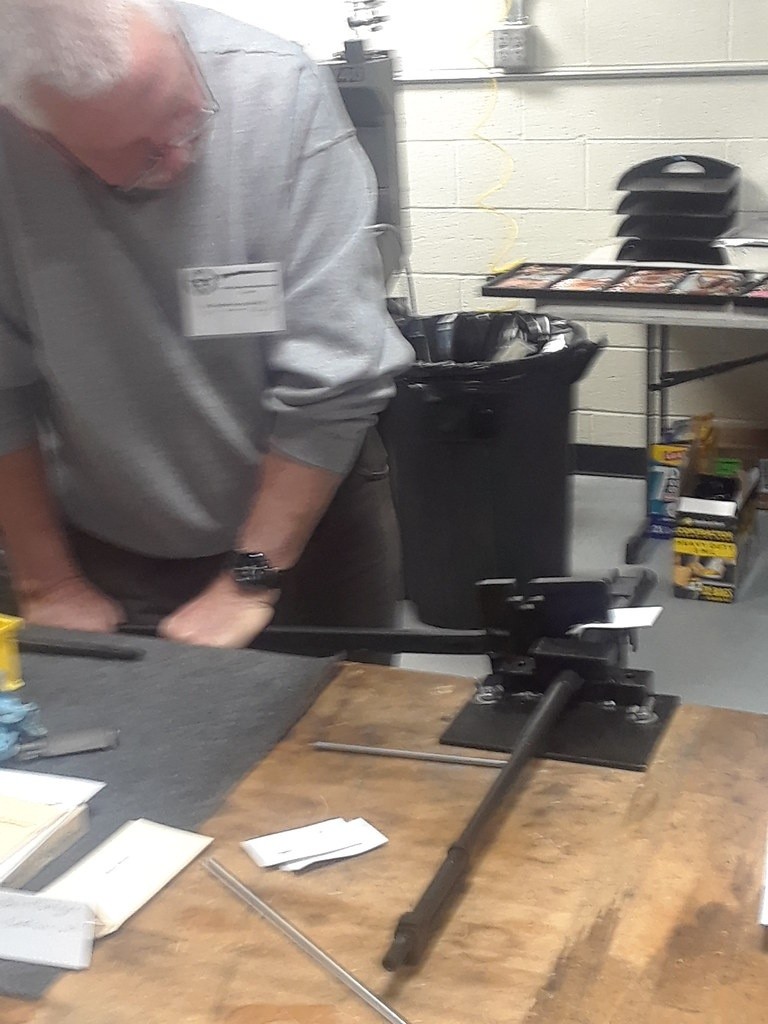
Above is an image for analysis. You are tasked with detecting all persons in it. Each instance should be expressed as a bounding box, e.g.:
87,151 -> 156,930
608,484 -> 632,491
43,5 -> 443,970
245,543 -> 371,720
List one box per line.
0,0 -> 418,671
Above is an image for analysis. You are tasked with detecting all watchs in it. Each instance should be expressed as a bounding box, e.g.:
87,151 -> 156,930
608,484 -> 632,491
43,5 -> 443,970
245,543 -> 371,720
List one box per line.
228,546 -> 295,591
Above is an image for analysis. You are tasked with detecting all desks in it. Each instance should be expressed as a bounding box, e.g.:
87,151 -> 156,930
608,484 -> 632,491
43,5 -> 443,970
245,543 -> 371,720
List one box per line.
526,237 -> 767,564
0,615 -> 768,1024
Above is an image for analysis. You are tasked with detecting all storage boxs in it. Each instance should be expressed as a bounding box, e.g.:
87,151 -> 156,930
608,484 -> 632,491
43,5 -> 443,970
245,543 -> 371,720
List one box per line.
668,428 -> 758,600
644,412 -> 719,539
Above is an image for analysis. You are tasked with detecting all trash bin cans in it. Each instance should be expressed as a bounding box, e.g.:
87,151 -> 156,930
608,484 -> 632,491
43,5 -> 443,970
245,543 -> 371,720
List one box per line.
371,310 -> 608,629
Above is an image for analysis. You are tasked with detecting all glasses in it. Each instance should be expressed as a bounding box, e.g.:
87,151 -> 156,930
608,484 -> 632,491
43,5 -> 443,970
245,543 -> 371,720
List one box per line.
39,22 -> 220,200
